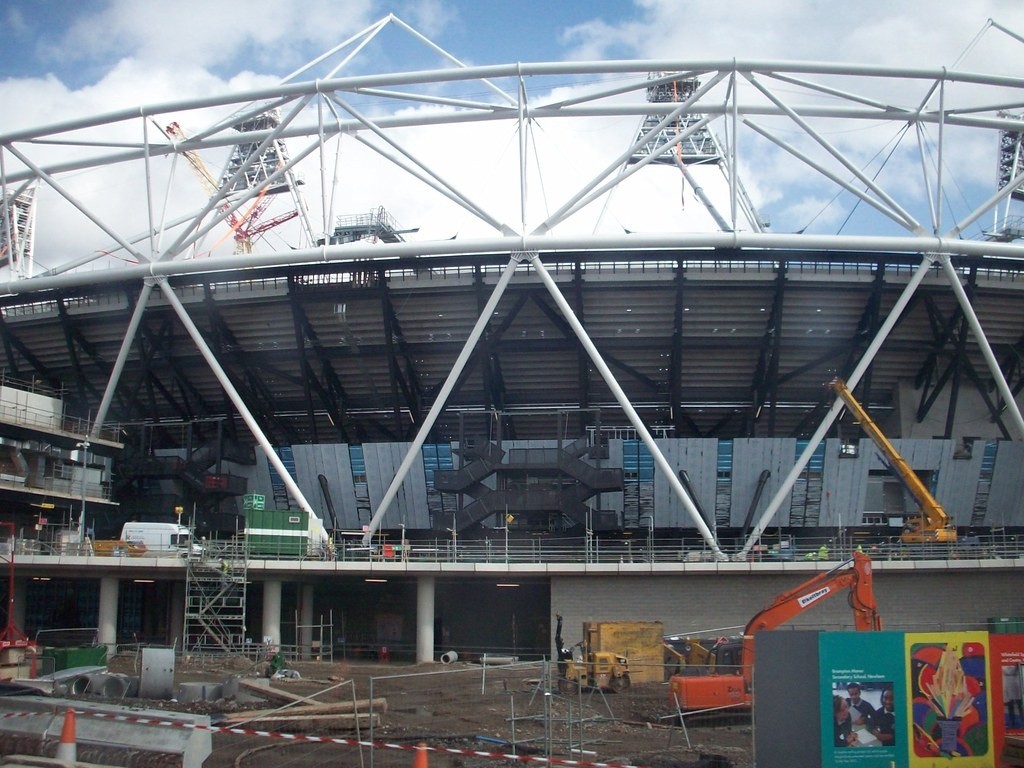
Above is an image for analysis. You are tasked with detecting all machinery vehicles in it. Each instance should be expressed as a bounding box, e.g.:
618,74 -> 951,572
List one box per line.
553,608 -> 718,696
829,373 -> 959,542
667,545 -> 885,726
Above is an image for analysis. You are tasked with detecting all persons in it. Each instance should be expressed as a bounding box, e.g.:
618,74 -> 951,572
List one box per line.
1001,665 -> 1024,727
818,543 -> 828,561
856,545 -> 863,554
844,683 -> 876,725
867,685 -> 896,746
805,552 -> 813,561
870,543 -> 878,553
834,694 -> 859,747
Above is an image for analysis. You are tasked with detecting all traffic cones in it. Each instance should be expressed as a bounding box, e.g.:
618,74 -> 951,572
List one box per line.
51,707 -> 76,764
413,743 -> 429,768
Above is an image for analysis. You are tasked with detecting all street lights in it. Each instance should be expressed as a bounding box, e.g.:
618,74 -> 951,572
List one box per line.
76,434 -> 91,556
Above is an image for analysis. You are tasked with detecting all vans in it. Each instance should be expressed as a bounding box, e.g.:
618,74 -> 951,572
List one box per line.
119,522 -> 208,559
